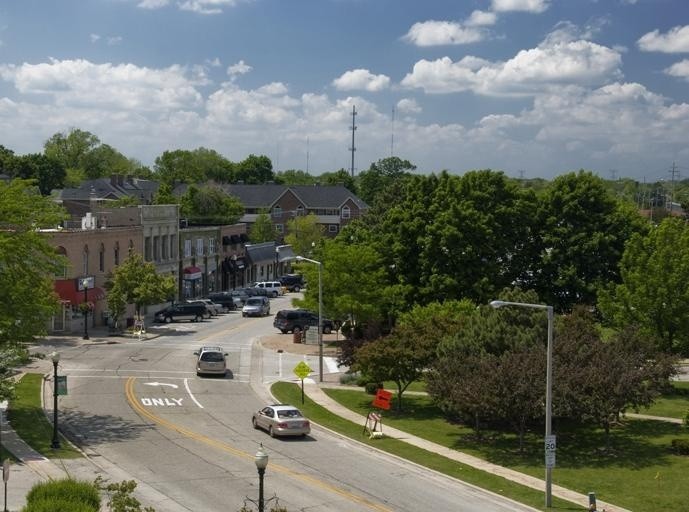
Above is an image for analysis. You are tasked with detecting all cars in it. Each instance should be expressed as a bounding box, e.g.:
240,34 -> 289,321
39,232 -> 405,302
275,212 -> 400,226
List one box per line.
252,402 -> 311,440
187,287 -> 270,319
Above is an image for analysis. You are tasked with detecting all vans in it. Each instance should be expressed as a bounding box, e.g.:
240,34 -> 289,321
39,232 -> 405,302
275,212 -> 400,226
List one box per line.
156,303 -> 207,323
194,346 -> 228,378
255,281 -> 283,298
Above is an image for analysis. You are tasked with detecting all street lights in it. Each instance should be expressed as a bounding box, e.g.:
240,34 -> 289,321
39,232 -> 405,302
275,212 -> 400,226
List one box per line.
48,351 -> 62,449
1,458 -> 10,512
241,441 -> 284,512
491,300 -> 554,509
83,279 -> 91,340
296,254 -> 325,382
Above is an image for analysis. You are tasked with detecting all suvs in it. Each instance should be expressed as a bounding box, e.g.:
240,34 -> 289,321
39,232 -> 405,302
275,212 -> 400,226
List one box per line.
276,276 -> 304,292
274,310 -> 333,335
